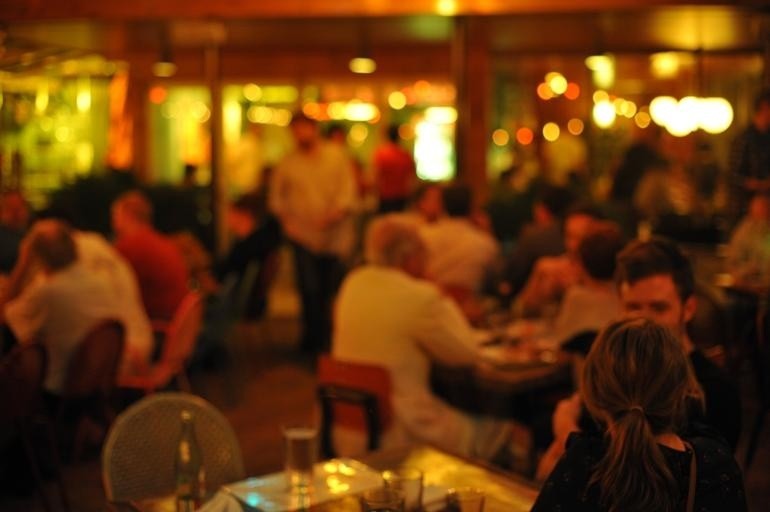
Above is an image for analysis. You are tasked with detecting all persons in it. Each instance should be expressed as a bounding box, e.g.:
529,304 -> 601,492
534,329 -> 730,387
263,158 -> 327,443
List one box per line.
334,107 -> 770,511
1,150 -> 282,438
267,111 -> 419,372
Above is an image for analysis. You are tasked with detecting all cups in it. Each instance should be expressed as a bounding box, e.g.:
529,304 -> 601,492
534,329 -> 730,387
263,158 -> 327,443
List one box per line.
360,464 -> 485,511
283,426 -> 321,488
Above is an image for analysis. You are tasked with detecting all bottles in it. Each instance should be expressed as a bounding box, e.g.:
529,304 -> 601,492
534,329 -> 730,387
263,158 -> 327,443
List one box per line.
171,407 -> 209,512
